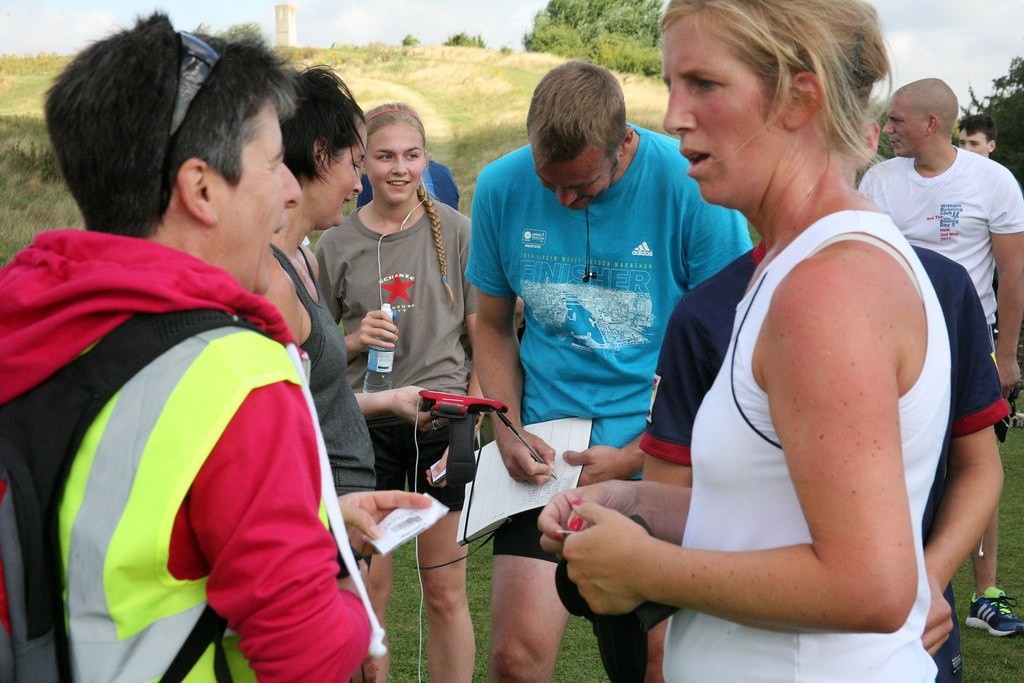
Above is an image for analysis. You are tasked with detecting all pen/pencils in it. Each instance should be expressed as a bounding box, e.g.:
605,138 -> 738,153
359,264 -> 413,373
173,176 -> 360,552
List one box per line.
494,409 -> 560,481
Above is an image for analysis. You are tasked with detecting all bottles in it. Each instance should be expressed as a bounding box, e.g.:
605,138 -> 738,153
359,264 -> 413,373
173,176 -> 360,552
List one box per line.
363,303 -> 395,394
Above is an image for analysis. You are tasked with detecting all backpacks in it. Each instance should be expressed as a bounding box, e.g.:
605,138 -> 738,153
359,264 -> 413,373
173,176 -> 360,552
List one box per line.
0,310 -> 227,683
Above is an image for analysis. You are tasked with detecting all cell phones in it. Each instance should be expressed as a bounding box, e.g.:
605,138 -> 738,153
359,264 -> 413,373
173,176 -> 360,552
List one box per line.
430,459 -> 446,485
631,514 -> 681,631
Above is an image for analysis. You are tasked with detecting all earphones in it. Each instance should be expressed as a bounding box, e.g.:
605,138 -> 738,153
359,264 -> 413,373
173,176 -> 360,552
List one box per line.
978,544 -> 985,558
583,270 -> 597,284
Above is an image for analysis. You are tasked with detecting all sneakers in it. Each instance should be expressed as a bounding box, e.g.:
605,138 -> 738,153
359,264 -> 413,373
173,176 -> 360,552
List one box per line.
963,585 -> 1024,638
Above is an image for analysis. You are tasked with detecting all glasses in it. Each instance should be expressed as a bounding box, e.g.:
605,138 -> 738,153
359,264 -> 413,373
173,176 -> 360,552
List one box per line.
168,29 -> 220,136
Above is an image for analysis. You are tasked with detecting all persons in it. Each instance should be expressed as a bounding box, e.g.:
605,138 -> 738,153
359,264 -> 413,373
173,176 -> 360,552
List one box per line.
313,102 -> 481,682
643,208 -> 1007,683
855,77 -> 1021,640
463,60 -> 756,681
536,0 -> 951,682
244,63 -> 393,682
354,153 -> 458,215
0,15 -> 424,682
957,113 -> 1023,428
